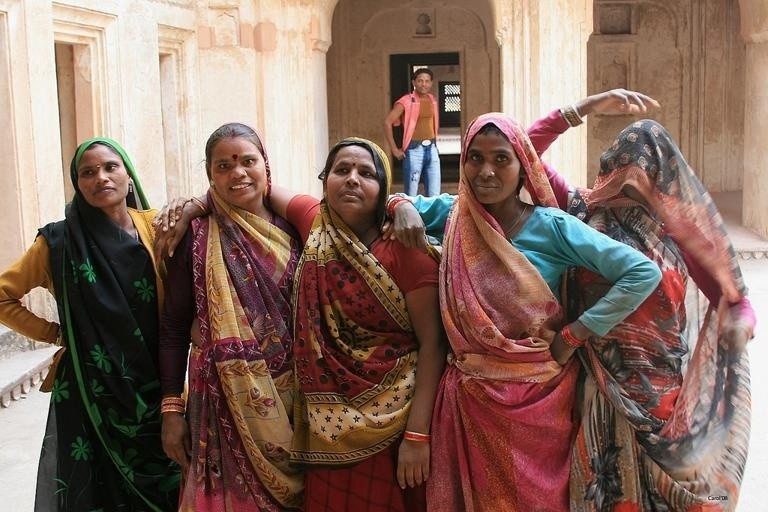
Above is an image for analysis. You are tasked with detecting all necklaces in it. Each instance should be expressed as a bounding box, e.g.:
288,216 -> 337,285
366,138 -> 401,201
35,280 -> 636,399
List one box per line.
136,230 -> 138,241
504,203 -> 528,235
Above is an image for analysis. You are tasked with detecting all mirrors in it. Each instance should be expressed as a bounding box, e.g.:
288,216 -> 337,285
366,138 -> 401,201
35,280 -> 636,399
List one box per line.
412,64 -> 461,127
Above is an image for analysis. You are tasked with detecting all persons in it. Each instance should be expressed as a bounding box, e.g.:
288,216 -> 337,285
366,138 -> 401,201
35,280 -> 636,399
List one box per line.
524,87 -> 756,512
383,112 -> 664,512
0,138 -> 185,512
152,137 -> 446,512
384,69 -> 441,197
157,123 -> 396,512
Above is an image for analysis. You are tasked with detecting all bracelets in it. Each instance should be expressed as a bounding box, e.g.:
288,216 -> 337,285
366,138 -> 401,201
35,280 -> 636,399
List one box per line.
561,325 -> 587,349
560,105 -> 583,128
191,196 -> 210,216
160,397 -> 186,414
386,196 -> 412,219
402,430 -> 431,442
55,326 -> 63,347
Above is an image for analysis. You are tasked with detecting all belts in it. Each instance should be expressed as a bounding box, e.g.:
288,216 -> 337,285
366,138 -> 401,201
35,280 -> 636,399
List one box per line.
410,139 -> 435,146
